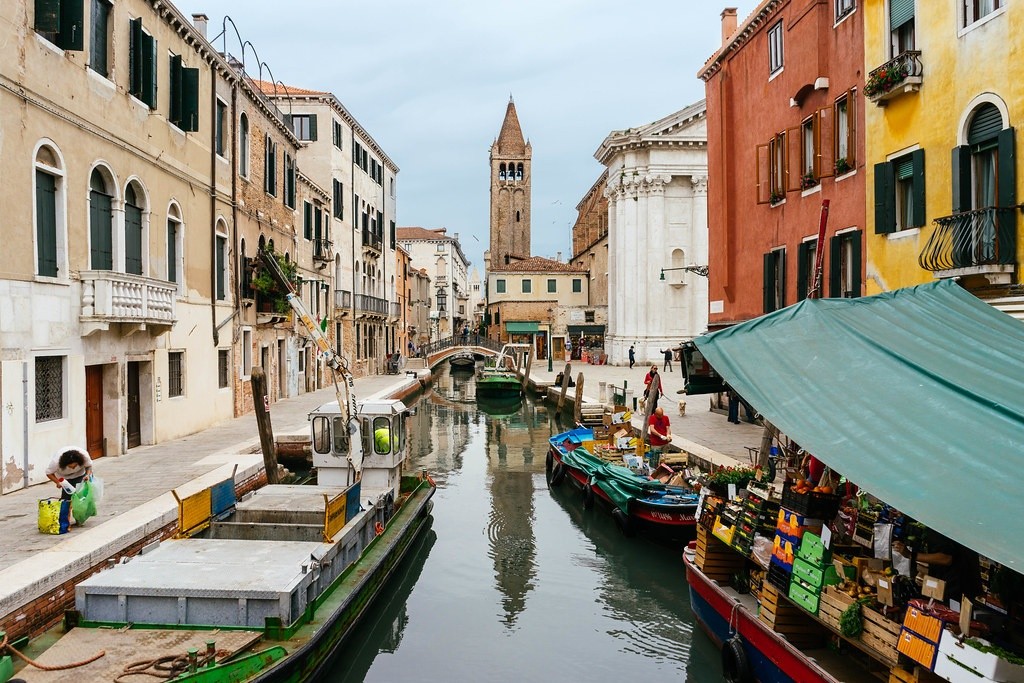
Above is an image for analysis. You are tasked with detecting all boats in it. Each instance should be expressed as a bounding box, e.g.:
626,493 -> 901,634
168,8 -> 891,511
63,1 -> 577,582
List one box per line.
682,539 -> 947,683
548,423 -> 697,540
474,344 -> 531,400
2,245 -> 438,683
474,388 -> 524,419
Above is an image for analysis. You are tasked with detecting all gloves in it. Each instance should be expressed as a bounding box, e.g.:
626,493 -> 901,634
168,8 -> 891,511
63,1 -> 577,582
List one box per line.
660,435 -> 667,441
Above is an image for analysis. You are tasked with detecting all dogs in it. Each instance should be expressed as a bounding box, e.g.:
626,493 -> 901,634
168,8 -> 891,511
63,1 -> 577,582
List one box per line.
639,398 -> 646,415
679,400 -> 687,417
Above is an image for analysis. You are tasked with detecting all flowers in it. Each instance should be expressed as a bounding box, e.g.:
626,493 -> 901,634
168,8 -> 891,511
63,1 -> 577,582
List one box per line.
769,191 -> 784,205
833,158 -> 849,174
798,172 -> 818,189
692,464 -> 769,491
863,61 -> 908,96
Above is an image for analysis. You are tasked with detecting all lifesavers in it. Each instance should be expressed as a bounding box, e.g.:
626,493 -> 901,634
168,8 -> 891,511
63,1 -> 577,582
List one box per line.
611,507 -> 637,539
720,637 -> 752,683
581,483 -> 595,508
546,451 -> 552,472
550,462 -> 567,486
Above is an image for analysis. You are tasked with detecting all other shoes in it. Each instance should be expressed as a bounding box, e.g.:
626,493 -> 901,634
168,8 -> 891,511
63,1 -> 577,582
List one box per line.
727,417 -> 741,424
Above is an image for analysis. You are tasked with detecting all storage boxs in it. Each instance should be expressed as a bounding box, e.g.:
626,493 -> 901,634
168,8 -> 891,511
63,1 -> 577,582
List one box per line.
696,480 -> 1024,683
583,405 -> 690,487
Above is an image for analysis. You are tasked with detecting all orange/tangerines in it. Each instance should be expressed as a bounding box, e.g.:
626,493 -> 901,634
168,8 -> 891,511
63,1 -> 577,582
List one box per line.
790,479 -> 833,495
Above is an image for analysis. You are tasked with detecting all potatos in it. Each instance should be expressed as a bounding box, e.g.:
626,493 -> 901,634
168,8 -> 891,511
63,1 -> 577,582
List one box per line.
879,566 -> 894,580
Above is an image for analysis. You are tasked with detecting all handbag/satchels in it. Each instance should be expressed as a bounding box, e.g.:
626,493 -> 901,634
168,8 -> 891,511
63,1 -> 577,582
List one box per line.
37,497 -> 71,535
71,478 -> 97,526
644,389 -> 649,397
76,470 -> 103,502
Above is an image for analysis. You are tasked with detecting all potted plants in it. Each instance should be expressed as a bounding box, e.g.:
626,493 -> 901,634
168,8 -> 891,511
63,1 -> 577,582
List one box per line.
250,248 -> 300,314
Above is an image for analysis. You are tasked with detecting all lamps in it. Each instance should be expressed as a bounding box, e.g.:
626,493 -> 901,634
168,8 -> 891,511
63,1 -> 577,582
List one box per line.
660,264 -> 709,282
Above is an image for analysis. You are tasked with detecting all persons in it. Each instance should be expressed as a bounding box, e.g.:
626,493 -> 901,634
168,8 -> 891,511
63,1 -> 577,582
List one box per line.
644,365 -> 663,414
455,323 -> 482,346
45,446 -> 93,534
649,407 -> 672,469
628,345 -> 636,369
408,340 -> 420,358
727,390 -> 755,424
564,341 -> 572,362
660,348 -> 673,372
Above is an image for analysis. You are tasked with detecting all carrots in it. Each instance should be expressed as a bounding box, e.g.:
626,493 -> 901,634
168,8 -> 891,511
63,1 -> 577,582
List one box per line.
837,577 -> 872,600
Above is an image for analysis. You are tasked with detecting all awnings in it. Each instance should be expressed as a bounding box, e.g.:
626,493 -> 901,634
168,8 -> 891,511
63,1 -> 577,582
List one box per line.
505,322 -> 538,334
679,279 -> 1024,575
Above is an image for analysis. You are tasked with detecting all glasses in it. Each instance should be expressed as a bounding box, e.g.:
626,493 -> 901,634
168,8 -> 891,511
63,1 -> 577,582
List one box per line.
652,368 -> 658,370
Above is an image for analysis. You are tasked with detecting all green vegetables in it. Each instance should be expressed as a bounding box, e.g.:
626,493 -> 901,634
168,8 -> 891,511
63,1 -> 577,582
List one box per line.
946,637 -> 1024,678
708,469 -> 772,491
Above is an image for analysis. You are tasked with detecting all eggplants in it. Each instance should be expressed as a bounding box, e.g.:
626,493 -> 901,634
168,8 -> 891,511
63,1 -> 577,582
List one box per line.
890,575 -> 922,605
